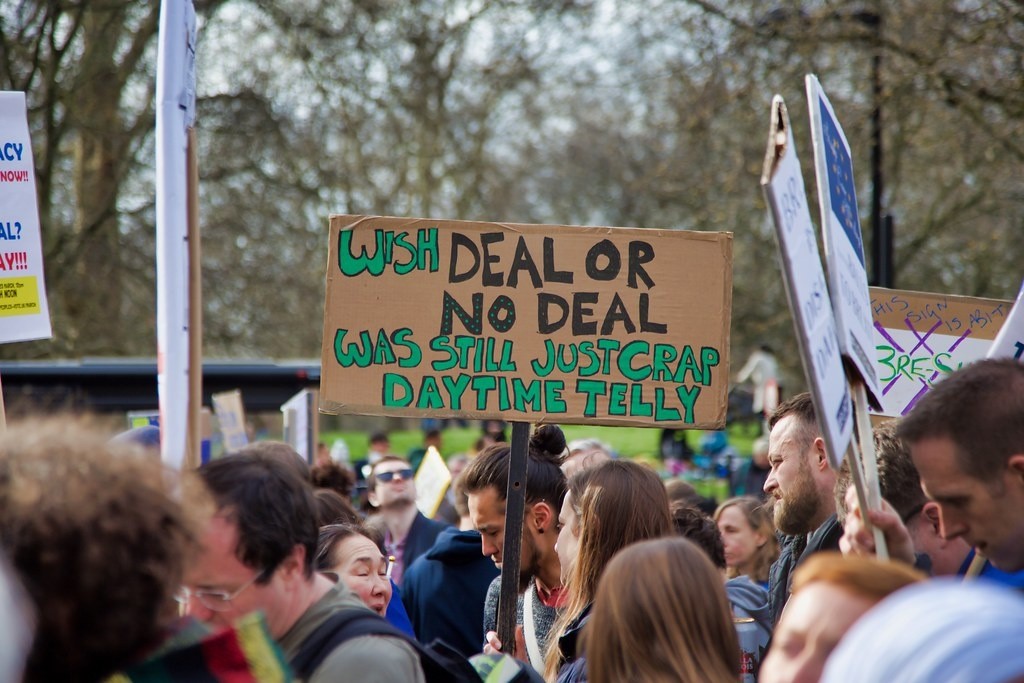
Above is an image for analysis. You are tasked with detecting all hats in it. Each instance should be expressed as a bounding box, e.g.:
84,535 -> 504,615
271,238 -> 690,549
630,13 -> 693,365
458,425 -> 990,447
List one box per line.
424,426 -> 438,439
368,431 -> 386,443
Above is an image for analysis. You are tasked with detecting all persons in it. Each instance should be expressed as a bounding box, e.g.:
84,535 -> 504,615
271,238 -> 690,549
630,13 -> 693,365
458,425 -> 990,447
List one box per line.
0,358 -> 1024,683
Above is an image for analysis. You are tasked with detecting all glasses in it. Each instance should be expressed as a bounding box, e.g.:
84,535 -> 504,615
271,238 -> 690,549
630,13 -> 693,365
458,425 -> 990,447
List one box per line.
376,469 -> 412,482
171,565 -> 268,613
